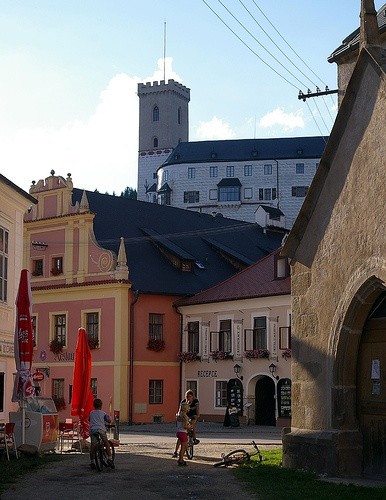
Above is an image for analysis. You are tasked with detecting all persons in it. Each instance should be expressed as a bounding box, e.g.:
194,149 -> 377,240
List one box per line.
87,398 -> 115,469
176,402 -> 190,466
171,389 -> 199,458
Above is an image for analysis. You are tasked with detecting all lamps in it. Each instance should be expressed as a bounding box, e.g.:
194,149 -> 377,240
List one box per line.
233,364 -> 244,380
269,363 -> 278,380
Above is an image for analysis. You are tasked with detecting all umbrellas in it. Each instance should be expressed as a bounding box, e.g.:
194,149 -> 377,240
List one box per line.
70,327 -> 95,453
10,268 -> 34,444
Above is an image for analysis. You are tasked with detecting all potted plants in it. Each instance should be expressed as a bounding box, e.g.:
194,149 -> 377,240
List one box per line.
50,340 -> 65,353
149,338 -> 165,352
89,336 -> 97,349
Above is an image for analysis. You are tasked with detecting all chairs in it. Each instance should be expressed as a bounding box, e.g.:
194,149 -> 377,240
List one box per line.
57,418 -> 86,455
0,422 -> 24,460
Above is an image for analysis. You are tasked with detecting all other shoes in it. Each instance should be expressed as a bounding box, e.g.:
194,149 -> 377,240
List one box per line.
89,463 -> 95,469
107,459 -> 115,469
172,452 -> 177,458
177,459 -> 188,466
193,437 -> 196,443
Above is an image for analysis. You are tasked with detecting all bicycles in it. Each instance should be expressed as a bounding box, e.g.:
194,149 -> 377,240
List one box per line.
213,441 -> 262,469
186,415 -> 197,460
90,424 -> 115,472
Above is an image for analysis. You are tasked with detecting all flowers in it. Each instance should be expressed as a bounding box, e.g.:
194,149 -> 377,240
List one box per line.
177,349 -> 272,360
282,348 -> 292,358
